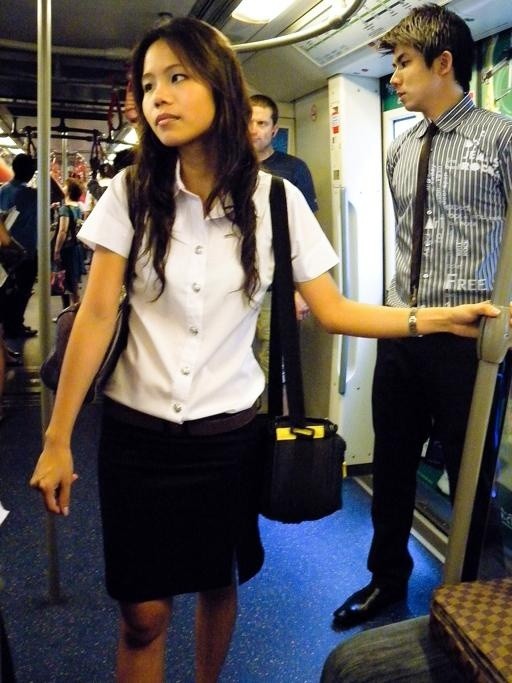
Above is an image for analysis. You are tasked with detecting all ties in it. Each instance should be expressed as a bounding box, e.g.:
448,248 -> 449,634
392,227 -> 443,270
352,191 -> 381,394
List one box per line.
410,126 -> 435,294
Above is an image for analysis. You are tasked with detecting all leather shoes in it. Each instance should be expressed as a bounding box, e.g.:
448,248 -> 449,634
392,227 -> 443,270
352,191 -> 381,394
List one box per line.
332,580 -> 408,621
3,325 -> 38,337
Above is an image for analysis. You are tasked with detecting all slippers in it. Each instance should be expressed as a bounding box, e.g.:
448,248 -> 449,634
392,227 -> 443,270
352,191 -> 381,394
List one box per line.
6,351 -> 23,366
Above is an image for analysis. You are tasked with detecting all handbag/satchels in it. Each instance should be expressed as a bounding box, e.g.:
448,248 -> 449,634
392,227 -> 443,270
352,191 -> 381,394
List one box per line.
430,575 -> 511,683
50,270 -> 66,295
260,413 -> 346,523
41,301 -> 132,406
